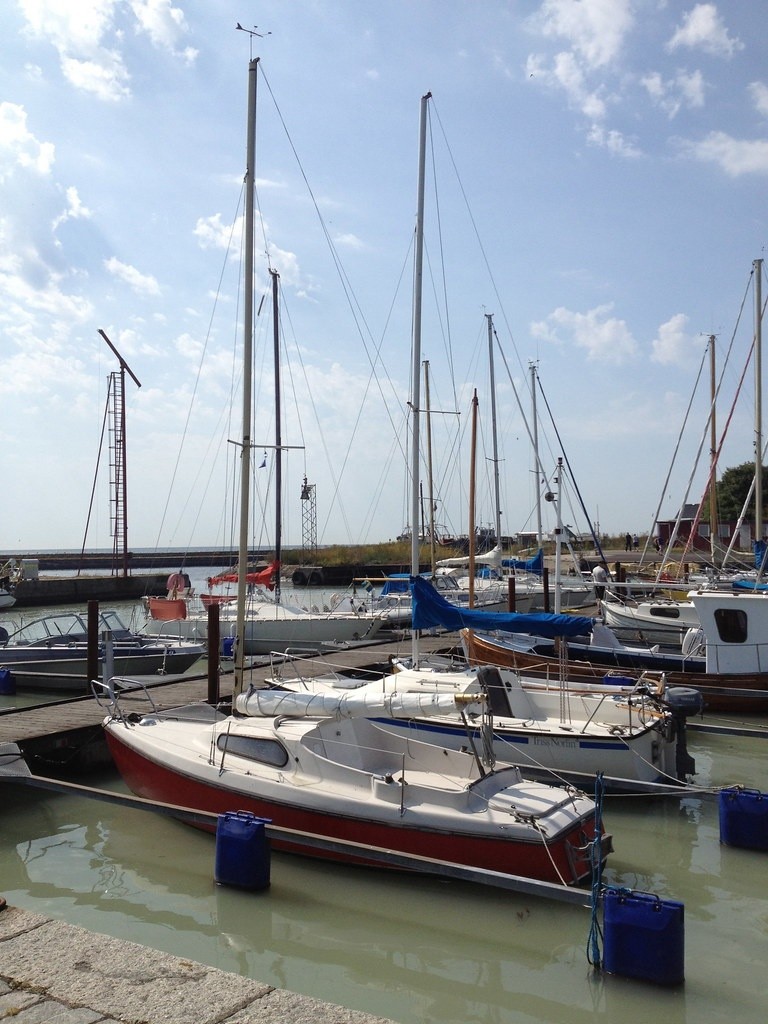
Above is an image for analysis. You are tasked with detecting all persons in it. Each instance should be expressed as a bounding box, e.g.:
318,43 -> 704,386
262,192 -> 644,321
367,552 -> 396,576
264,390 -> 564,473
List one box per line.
632,534 -> 639,552
591,559 -> 607,614
653,537 -> 661,553
625,532 -> 632,553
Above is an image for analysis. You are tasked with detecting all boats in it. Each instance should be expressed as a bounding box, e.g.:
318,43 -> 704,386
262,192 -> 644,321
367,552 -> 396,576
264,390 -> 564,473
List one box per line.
373,566 -> 588,612
458,628 -> 768,713
199,593 -> 237,611
144,595 -> 187,621
1,611 -> 206,694
236,666 -> 698,808
104,712 -> 613,911
610,571 -> 768,604
601,599 -> 702,646
390,656 -> 667,701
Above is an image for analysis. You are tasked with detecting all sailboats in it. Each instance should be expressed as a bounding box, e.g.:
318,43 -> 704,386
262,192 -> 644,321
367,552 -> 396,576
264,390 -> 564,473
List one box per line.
145,265 -> 388,655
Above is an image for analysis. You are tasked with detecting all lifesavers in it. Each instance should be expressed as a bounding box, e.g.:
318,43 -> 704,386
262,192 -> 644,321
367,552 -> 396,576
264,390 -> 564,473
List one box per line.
166,573 -> 185,592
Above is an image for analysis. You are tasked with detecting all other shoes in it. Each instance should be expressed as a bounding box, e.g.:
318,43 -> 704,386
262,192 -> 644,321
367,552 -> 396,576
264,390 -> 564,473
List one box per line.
597,611 -> 602,616
